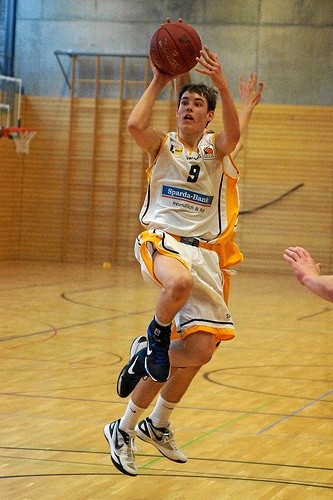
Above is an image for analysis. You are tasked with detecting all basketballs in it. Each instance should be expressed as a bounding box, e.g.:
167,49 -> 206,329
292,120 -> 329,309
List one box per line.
149,22 -> 203,76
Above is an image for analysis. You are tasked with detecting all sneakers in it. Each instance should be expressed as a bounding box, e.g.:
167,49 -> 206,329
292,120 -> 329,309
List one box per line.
144,318 -> 171,382
103,419 -> 138,476
134,417 -> 188,463
117,335 -> 150,398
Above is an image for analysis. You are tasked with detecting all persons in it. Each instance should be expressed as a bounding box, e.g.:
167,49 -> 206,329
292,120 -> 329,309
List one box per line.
103,17 -> 265,478
283,247 -> 333,302
117,24 -> 240,399
